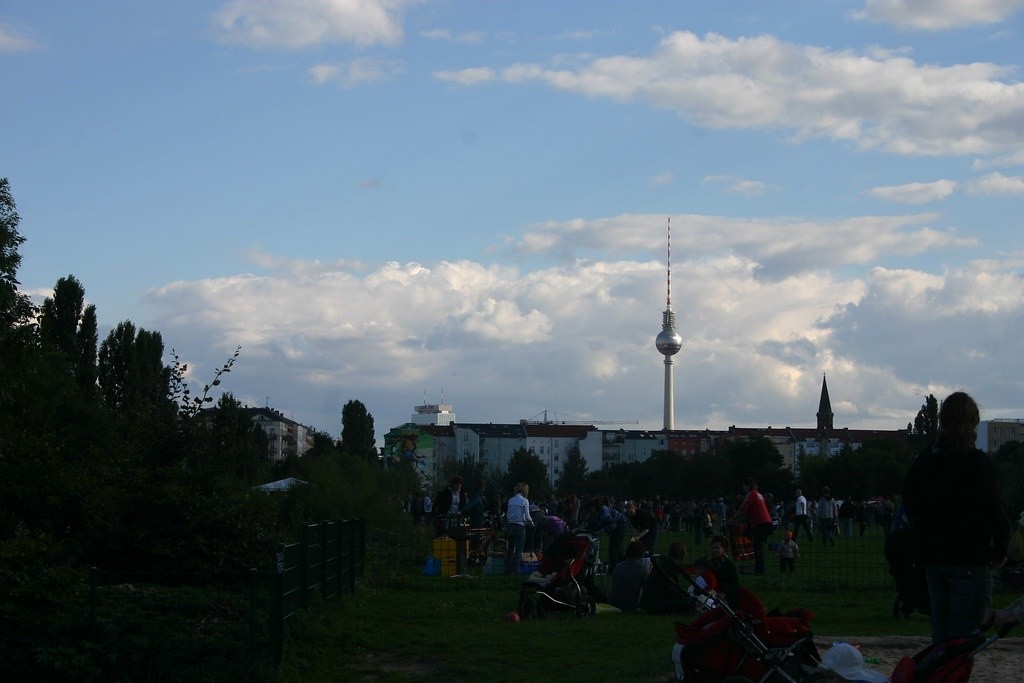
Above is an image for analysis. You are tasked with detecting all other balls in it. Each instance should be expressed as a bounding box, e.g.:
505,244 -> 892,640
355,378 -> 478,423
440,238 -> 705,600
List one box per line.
506,611 -> 520,624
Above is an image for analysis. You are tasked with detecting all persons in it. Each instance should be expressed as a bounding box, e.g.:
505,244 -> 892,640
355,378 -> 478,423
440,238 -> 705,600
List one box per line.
791,489 -> 814,542
901,394 -> 1010,644
504,482 -> 535,575
551,495 -> 717,532
625,502 -> 657,557
642,542 -> 697,615
717,497 -> 726,527
459,479 -> 488,550
523,504 -> 569,553
435,477 -> 465,536
694,535 -> 740,605
726,478 -> 773,575
816,487 -> 838,547
693,504 -> 705,545
401,491 -> 443,524
984,596 -> 1024,631
776,531 -> 800,577
607,541 -> 650,612
763,494 -> 893,538
588,497 -> 627,575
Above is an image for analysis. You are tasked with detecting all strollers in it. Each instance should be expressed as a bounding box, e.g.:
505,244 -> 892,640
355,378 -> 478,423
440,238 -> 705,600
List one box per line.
883,528 -> 925,622
885,609 -> 1015,683
517,525 -> 596,621
674,573 -> 827,683
570,525 -> 604,575
717,518 -> 757,572
663,517 -> 673,531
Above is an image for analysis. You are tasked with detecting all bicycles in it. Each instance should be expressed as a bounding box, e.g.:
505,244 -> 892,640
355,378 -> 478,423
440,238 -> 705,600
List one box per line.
461,511 -> 509,555
